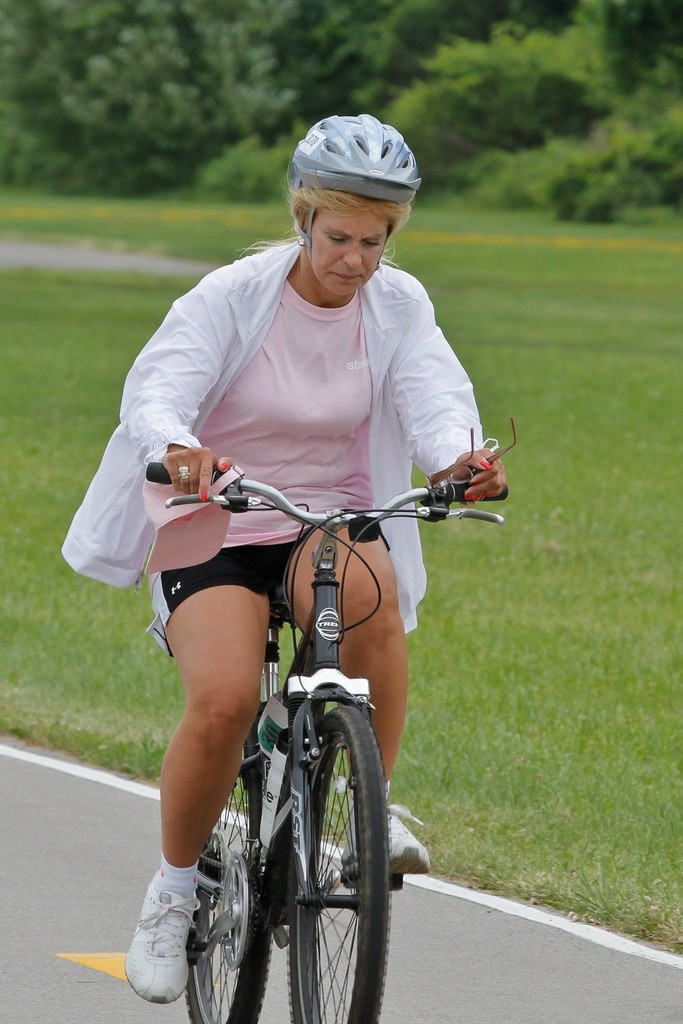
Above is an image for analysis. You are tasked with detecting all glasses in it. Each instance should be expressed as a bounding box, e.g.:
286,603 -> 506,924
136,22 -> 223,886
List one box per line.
425,415 -> 516,488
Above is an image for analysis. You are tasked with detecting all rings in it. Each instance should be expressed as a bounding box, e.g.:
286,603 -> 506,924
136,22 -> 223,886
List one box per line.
178,467 -> 189,479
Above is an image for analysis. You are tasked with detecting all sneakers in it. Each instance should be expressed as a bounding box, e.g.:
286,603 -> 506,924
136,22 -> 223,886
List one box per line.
125,879 -> 202,1004
340,808 -> 432,874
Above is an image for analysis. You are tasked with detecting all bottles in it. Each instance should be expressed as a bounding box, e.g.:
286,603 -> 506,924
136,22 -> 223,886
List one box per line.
259,728 -> 289,849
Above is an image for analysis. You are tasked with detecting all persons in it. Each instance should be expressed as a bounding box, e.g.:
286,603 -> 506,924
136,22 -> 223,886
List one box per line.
59,111 -> 507,1006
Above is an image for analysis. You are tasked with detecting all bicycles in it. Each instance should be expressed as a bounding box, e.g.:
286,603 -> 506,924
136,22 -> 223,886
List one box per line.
142,462 -> 509,1024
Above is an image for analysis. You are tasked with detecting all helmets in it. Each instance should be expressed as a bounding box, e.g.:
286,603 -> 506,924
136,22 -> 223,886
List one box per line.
291,112 -> 421,206
